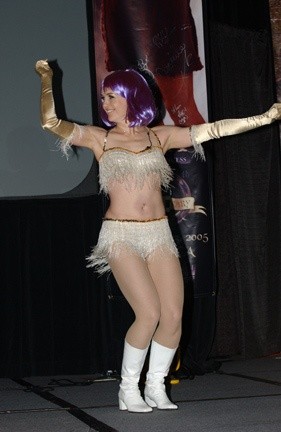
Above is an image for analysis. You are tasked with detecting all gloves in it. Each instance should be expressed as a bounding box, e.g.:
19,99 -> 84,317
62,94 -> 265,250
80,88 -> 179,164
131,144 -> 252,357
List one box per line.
190,102 -> 281,147
34,60 -> 74,140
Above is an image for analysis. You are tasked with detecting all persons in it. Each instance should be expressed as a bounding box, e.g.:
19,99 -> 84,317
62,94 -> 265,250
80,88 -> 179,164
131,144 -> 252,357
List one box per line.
34,59 -> 280,412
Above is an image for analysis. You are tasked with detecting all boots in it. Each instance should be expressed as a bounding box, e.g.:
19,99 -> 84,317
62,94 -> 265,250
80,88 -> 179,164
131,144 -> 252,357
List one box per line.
144,340 -> 179,410
117,338 -> 153,413
